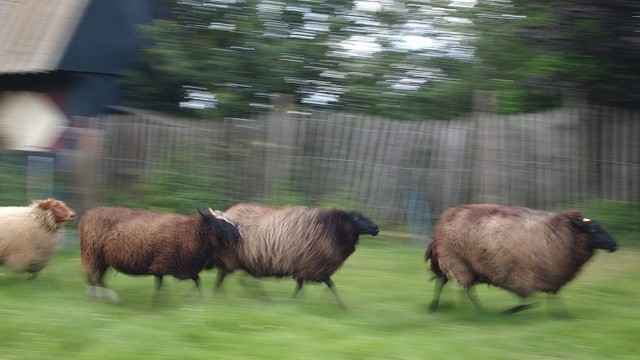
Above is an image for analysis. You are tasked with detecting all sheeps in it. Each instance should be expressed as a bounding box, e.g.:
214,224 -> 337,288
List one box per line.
0,197 -> 77,283
425,202 -> 617,324
211,199 -> 380,315
73,200 -> 243,308
